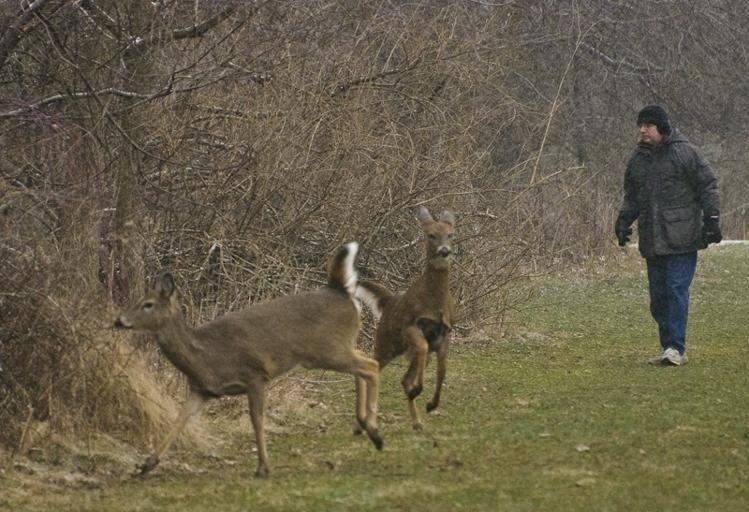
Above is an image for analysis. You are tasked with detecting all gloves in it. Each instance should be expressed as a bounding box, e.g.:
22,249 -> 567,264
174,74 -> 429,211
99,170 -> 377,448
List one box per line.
616,221 -> 632,246
701,215 -> 722,243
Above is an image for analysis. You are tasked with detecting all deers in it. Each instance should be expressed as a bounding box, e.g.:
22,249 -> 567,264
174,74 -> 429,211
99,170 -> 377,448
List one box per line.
354,205 -> 455,431
113,241 -> 384,482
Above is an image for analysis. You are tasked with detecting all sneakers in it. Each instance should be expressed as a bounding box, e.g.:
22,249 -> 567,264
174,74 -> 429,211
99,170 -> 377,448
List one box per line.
648,347 -> 689,366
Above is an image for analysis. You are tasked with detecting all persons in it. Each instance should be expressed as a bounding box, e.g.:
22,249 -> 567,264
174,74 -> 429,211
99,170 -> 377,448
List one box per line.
614,104 -> 722,366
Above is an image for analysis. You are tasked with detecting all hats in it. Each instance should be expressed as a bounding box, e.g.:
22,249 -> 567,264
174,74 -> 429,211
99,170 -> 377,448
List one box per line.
638,106 -> 671,134
